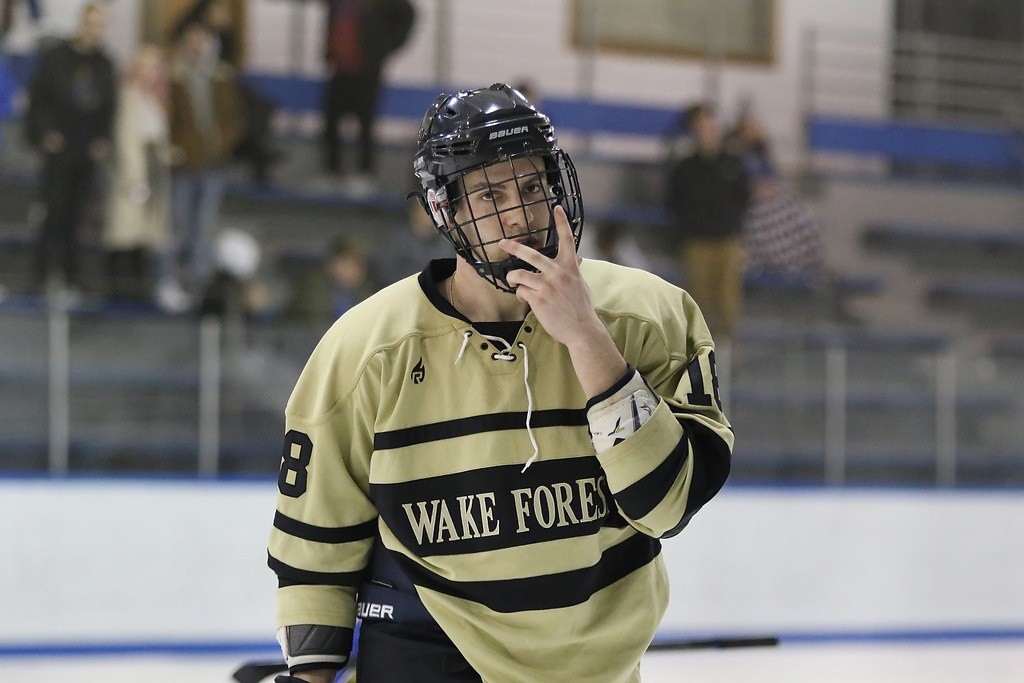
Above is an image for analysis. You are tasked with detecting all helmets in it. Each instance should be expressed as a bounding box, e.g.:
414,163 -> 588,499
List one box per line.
414,80 -> 585,292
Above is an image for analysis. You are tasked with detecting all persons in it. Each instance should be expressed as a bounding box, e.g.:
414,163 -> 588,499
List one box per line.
0,0 -> 417,327
661,98 -> 794,333
265,84 -> 735,683
